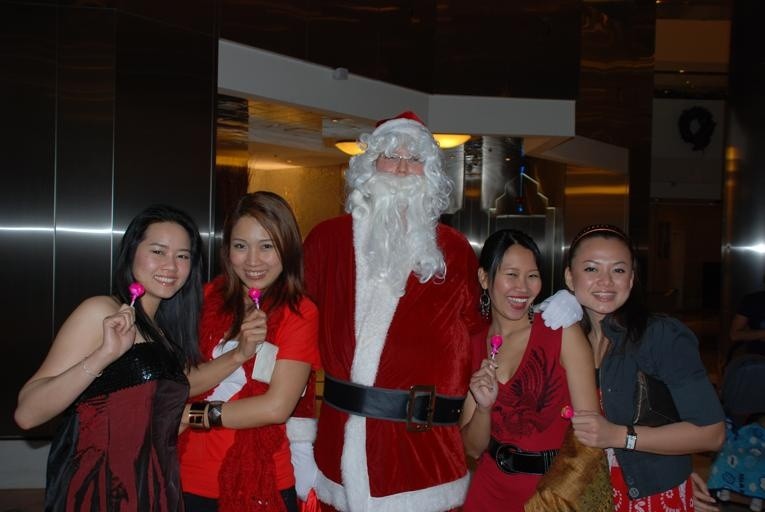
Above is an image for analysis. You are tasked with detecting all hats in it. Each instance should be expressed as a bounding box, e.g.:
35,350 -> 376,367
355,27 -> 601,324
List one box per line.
374,112 -> 433,136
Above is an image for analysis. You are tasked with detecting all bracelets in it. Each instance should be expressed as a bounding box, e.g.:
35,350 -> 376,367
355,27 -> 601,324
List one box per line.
188,402 -> 211,431
82,356 -> 103,377
207,401 -> 224,427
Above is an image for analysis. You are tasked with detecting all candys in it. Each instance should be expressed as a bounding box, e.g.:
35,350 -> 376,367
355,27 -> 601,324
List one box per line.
248,287 -> 261,311
561,406 -> 575,419
489,335 -> 502,368
129,282 -> 144,307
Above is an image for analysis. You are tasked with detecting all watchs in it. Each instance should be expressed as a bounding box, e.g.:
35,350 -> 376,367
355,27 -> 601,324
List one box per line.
624,425 -> 637,451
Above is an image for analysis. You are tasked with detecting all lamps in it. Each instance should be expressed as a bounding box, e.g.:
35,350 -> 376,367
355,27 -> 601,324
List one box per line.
333,138 -> 368,156
432,133 -> 471,149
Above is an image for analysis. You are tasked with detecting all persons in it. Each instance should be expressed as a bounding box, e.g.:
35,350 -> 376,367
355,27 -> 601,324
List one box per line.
285,111 -> 587,511
564,224 -> 727,512
461,228 -> 601,512
13,203 -> 205,511
727,269 -> 765,363
176,191 -> 322,511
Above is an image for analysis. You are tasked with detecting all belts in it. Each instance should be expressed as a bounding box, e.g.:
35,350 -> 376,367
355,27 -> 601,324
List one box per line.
485,438 -> 563,474
321,376 -> 463,428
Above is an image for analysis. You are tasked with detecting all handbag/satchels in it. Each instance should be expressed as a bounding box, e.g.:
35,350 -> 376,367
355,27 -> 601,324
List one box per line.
633,372 -> 680,426
